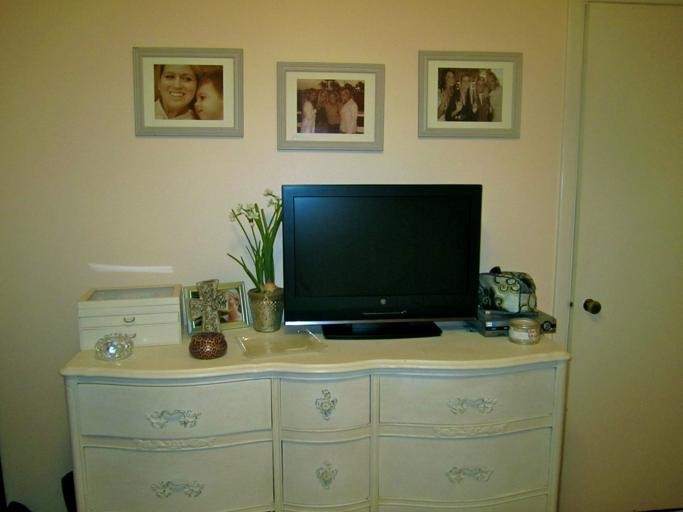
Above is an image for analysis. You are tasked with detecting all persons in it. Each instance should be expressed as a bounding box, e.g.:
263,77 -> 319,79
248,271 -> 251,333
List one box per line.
338,89 -> 358,134
314,89 -> 329,133
445,75 -> 474,122
300,89 -> 320,133
192,66 -> 223,120
438,70 -> 458,121
219,288 -> 244,323
154,64 -> 203,121
470,78 -> 490,122
325,92 -> 340,133
487,73 -> 502,121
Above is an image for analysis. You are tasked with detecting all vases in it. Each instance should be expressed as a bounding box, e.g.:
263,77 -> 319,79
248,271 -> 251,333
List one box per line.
246,287 -> 283,333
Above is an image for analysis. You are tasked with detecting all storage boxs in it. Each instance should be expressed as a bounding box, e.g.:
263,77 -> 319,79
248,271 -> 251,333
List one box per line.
75,283 -> 184,350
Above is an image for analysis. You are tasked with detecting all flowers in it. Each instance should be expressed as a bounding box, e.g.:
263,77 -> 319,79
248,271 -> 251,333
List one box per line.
225,188 -> 284,297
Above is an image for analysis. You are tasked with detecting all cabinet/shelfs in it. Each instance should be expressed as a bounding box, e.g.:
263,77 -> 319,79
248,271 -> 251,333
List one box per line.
55,324 -> 573,512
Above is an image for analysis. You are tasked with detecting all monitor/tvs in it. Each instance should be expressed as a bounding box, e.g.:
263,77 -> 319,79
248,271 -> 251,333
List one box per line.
281,183 -> 481,341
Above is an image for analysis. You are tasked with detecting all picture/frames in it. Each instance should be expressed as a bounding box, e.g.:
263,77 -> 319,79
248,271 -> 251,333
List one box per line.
130,43 -> 245,139
416,49 -> 525,143
182,278 -> 250,337
272,59 -> 386,153
